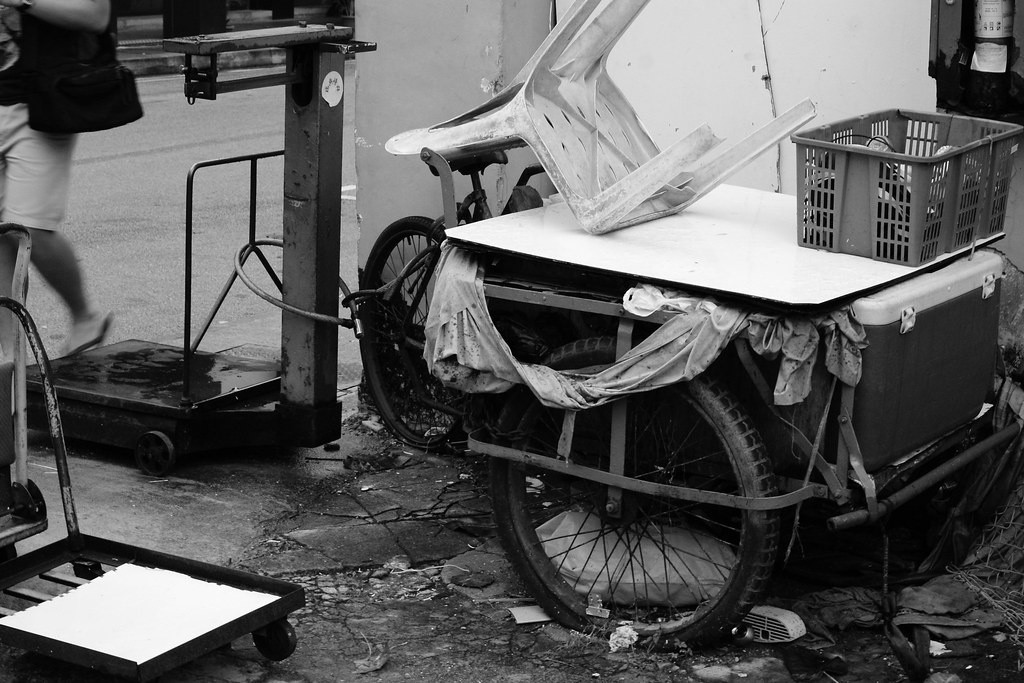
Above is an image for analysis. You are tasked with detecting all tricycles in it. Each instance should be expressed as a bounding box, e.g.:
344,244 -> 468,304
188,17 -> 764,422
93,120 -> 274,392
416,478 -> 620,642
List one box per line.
355,147 -> 1024,654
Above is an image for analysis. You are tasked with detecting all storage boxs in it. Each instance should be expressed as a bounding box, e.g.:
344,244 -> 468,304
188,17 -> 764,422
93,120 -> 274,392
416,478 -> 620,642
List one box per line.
850,250 -> 1007,476
789,108 -> 1024,269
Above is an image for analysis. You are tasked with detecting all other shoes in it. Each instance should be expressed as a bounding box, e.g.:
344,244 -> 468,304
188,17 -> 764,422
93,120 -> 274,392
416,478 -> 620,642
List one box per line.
62,315 -> 110,355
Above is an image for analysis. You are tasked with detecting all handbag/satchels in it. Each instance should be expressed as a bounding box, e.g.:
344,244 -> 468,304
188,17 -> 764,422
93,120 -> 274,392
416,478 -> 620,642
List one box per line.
19,14 -> 144,133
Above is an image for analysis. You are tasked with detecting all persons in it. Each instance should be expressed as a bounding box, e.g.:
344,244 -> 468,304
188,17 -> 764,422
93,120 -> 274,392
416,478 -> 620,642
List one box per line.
0,1 -> 110,415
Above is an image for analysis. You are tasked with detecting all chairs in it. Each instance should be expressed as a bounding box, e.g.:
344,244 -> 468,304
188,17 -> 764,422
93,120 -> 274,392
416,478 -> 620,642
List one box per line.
383,0 -> 818,237
